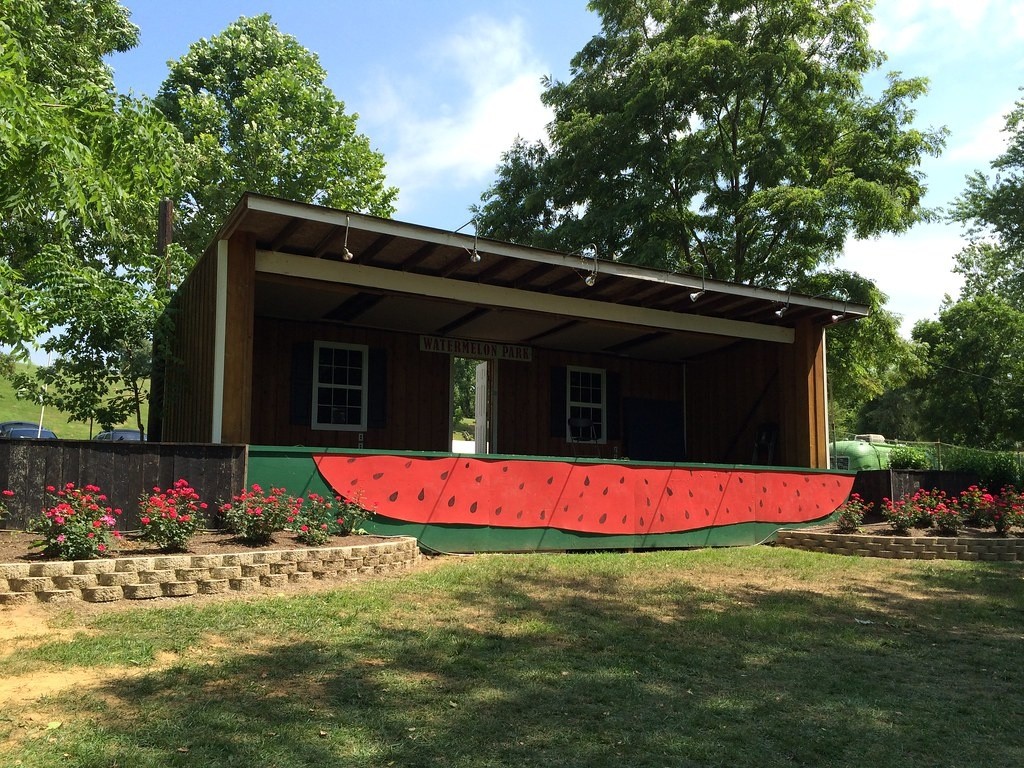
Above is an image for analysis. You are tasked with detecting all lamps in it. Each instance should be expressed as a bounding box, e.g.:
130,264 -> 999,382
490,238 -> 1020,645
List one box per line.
758,279 -> 793,319
342,213 -> 353,261
669,263 -> 706,303
564,243 -> 599,286
811,288 -> 849,323
453,218 -> 481,262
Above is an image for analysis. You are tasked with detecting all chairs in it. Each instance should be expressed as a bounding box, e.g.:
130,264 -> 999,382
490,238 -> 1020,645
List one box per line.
752,422 -> 779,465
568,418 -> 601,458
